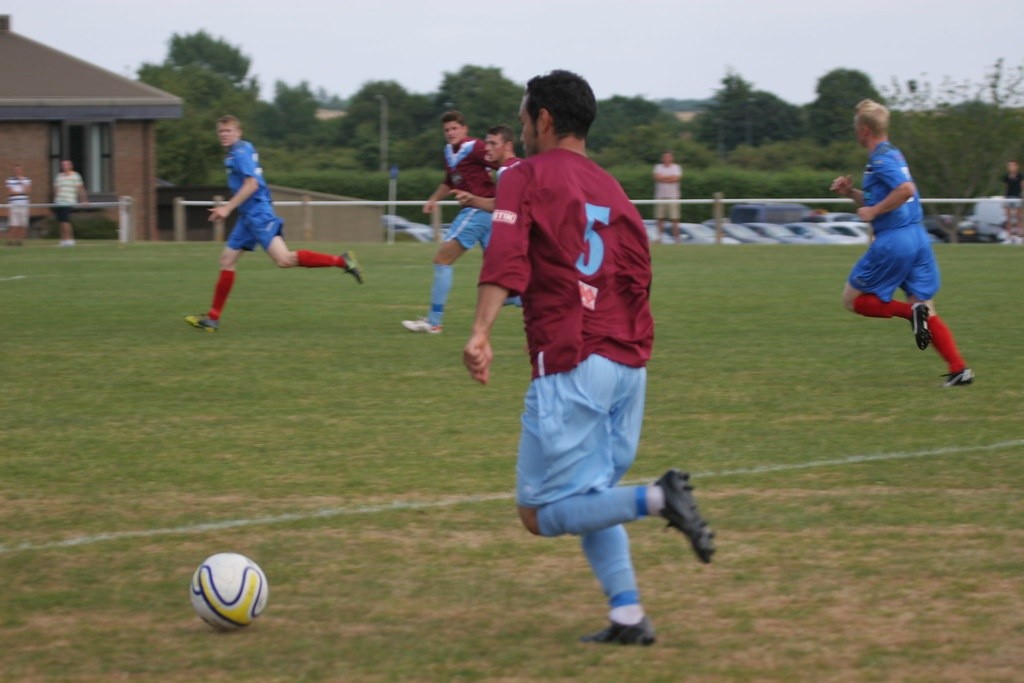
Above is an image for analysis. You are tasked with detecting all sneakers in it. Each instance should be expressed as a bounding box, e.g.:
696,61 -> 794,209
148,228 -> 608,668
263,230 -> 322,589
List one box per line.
184,315 -> 220,333
941,368 -> 974,388
909,300 -> 931,350
341,251 -> 366,284
578,613 -> 656,647
655,467 -> 714,563
401,319 -> 444,334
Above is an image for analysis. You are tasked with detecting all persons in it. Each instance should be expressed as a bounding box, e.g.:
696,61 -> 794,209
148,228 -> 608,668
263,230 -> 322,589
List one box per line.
1002,160 -> 1024,245
450,127 -> 523,213
465,70 -> 715,645
6,164 -> 31,246
831,101 -> 974,386
185,115 -> 364,331
52,158 -> 83,246
652,152 -> 682,241
401,111 -> 523,333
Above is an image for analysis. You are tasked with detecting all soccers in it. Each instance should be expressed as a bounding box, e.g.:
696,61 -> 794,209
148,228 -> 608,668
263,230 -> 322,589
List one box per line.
189,553 -> 267,631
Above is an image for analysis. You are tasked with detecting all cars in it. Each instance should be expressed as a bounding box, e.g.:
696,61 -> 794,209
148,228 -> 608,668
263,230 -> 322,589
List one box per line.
926,215 -> 1013,246
383,214 -> 452,243
635,200 -> 876,246
